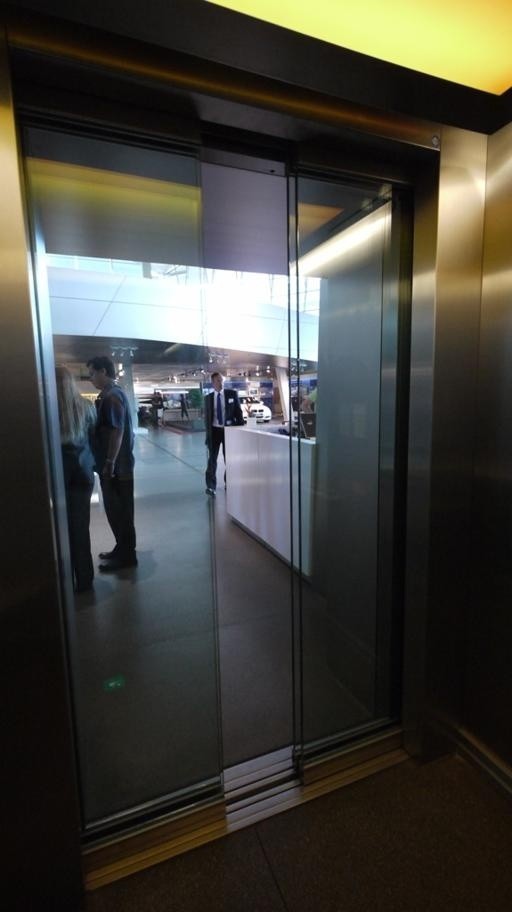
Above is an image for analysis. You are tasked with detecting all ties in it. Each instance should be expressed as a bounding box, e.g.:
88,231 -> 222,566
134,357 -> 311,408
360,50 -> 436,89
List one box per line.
216,392 -> 223,425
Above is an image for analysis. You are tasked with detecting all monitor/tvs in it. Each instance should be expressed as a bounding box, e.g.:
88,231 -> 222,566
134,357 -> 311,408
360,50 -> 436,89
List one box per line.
299,414 -> 315,437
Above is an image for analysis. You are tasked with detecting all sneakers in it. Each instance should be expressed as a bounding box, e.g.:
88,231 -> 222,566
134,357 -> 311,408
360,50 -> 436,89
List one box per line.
99,546 -> 138,571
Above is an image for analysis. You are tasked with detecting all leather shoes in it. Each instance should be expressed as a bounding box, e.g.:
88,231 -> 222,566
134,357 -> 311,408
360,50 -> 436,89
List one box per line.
205,487 -> 217,496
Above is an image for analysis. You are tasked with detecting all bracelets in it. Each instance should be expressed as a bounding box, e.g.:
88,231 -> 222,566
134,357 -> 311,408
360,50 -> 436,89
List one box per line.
103,457 -> 116,465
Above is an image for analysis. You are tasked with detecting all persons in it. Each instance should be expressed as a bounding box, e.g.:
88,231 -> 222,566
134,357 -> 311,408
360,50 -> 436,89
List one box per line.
203,370 -> 246,498
83,355 -> 138,573
137,389 -> 192,429
300,383 -> 317,414
54,365 -> 99,598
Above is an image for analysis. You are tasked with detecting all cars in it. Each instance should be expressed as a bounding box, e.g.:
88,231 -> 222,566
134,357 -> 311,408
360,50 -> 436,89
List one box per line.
238,396 -> 272,423
